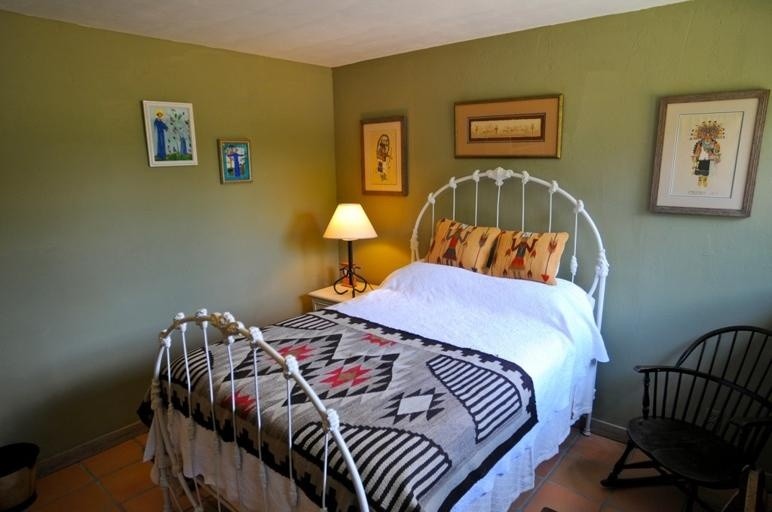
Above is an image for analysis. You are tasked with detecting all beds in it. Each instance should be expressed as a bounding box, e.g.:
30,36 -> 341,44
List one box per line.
136,165 -> 610,512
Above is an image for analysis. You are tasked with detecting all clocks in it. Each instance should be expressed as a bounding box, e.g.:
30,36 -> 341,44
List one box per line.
339,261 -> 359,288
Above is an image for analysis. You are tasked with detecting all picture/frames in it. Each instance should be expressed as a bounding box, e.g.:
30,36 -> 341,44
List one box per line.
453,93 -> 562,159
360,115 -> 409,196
218,137 -> 253,184
142,99 -> 198,167
646,88 -> 769,218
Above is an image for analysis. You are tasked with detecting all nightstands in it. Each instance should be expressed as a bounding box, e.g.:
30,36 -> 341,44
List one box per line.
307,283 -> 382,312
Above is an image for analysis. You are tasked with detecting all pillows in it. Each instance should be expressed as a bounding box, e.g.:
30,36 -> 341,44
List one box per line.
380,261 -> 609,364
422,217 -> 500,272
489,231 -> 568,285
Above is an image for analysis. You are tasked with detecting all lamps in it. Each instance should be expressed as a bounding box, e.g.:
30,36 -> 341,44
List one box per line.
321,204 -> 379,298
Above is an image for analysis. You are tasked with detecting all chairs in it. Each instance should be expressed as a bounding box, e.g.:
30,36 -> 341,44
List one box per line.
600,325 -> 772,512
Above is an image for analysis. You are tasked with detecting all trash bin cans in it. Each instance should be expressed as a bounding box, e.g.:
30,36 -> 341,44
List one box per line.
0,442 -> 39,512
